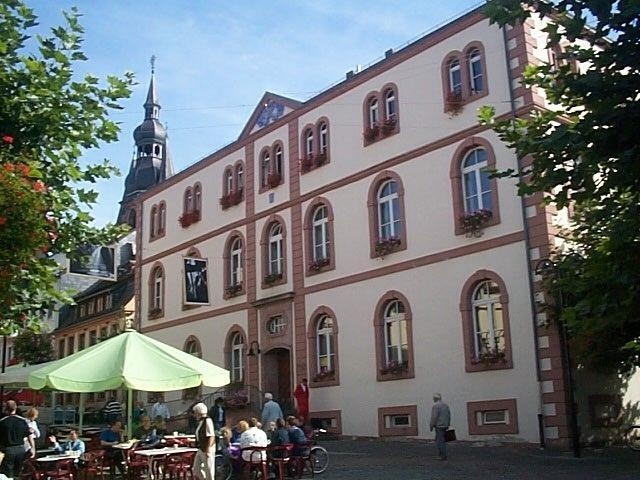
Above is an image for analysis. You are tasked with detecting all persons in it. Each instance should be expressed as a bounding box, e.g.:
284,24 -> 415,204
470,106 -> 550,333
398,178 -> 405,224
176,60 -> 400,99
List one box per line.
191,402 -> 216,480
0,399 -> 36,480
24,407 -> 41,459
208,396 -> 226,429
293,378 -> 309,425
98,396 -> 170,480
48,429 -> 86,470
217,415 -> 307,480
261,393 -> 283,424
428,392 -> 451,461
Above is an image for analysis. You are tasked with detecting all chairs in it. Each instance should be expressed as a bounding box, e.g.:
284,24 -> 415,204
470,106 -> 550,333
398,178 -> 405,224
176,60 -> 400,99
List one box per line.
25,424 -> 318,480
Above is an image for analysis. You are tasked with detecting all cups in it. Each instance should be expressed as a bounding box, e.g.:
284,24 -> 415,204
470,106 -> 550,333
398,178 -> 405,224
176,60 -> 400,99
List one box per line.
174,443 -> 179,449
172,431 -> 179,437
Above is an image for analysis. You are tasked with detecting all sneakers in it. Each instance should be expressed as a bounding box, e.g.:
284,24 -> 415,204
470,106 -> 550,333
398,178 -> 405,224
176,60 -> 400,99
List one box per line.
432,454 -> 446,460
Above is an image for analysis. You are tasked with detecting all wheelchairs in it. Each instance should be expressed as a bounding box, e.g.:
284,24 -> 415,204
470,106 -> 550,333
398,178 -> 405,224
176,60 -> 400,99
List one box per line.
215,435 -> 241,480
304,428 -> 329,474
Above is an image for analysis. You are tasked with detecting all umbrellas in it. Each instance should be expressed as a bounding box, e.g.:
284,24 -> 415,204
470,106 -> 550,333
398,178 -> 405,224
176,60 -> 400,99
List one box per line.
28,318 -> 232,442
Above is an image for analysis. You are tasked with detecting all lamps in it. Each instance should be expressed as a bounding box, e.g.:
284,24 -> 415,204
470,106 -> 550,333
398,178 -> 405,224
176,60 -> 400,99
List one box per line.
247,341 -> 261,356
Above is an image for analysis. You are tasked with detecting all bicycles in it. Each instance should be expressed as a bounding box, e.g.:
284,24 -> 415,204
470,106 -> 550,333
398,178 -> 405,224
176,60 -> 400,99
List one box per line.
625,424 -> 640,451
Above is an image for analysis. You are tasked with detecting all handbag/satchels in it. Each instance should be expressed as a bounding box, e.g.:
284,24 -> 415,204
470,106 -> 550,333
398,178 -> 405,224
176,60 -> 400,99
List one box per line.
444,426 -> 456,443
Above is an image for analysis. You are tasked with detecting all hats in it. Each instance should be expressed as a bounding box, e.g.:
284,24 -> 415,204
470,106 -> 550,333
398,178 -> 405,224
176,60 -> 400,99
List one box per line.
194,402 -> 209,414
264,393 -> 274,399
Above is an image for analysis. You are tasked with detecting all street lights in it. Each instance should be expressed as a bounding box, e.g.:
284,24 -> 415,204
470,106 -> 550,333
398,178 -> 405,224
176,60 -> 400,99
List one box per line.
0,326 -> 19,420
534,252 -> 585,458
